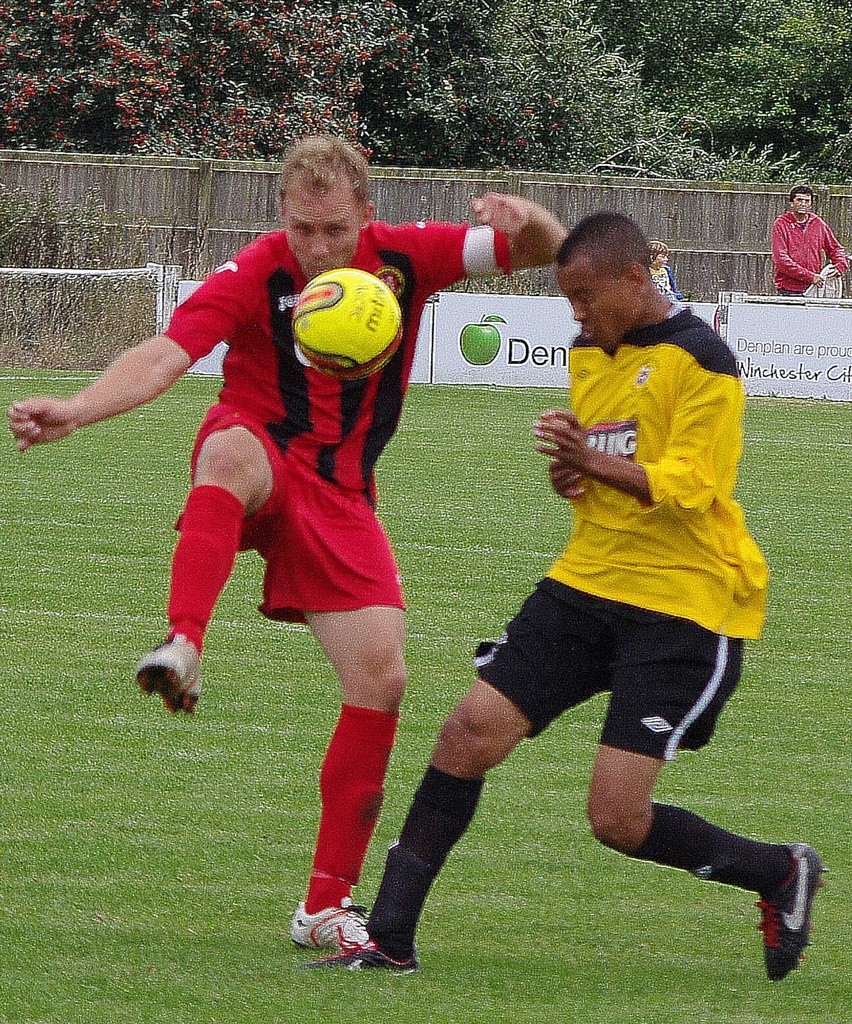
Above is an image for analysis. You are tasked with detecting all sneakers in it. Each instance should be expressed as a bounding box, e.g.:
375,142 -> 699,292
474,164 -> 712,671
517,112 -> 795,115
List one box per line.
298,940 -> 418,977
754,842 -> 824,982
135,633 -> 203,714
289,897 -> 370,949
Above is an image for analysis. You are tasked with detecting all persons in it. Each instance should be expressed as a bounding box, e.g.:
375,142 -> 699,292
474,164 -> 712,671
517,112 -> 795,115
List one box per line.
8,133 -> 567,953
647,240 -> 683,302
302,211 -> 824,982
771,185 -> 847,298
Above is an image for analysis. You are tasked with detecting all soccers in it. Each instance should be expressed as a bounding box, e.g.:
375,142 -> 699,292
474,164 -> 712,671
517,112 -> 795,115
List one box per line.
289,267 -> 406,381
